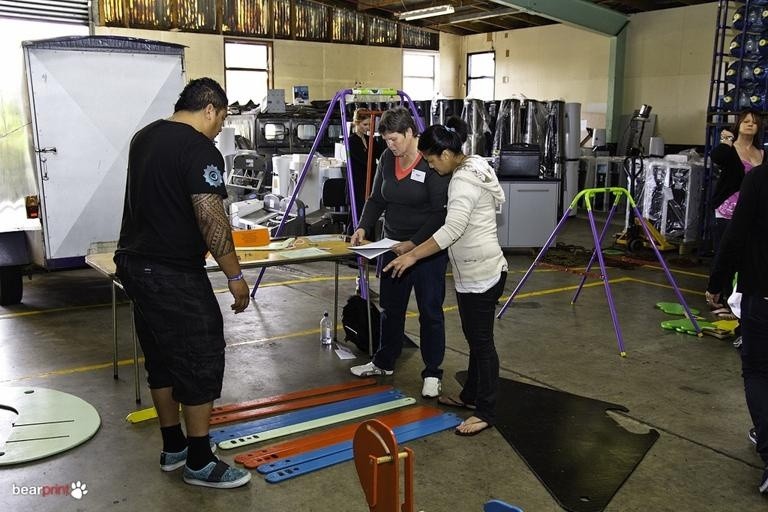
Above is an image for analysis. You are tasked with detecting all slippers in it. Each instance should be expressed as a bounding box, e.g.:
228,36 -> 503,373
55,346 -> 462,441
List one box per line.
455,415 -> 494,436
438,394 -> 476,411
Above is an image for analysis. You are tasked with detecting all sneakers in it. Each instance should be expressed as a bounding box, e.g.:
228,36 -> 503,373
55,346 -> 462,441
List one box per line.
183,455 -> 251,489
350,362 -> 394,377
160,441 -> 217,473
422,377 -> 445,398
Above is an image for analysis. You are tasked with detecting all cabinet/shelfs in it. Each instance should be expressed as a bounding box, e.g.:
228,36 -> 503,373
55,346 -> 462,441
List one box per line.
491,176 -> 562,259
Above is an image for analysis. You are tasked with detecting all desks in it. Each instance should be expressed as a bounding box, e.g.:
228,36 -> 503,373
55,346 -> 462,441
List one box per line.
79,230 -> 380,405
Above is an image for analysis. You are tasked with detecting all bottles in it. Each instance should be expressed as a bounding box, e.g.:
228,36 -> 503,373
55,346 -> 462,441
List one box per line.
319,311 -> 333,345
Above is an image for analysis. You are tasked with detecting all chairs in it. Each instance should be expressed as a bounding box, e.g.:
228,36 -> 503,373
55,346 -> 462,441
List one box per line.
320,174 -> 353,233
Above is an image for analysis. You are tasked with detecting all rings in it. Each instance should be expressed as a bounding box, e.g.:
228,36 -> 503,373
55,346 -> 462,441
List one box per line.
706,300 -> 709,303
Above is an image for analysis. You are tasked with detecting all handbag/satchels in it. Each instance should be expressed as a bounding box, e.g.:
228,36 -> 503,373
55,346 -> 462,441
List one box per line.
342,295 -> 380,355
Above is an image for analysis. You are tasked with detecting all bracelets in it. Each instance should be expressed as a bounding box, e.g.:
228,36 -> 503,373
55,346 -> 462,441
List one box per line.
718,136 -> 735,140
227,272 -> 244,283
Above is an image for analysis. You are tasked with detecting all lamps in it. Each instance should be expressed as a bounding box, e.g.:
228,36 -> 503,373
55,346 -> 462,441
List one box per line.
396,2 -> 454,21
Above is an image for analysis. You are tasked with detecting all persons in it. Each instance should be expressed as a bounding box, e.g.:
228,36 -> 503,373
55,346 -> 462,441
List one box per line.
706,165 -> 768,494
350,108 -> 449,397
113,77 -> 252,490
345,106 -> 380,269
710,108 -> 768,320
383,118 -> 508,436
